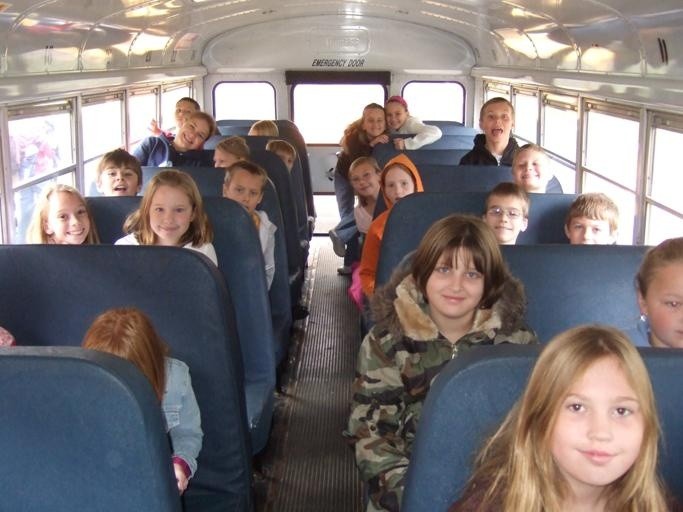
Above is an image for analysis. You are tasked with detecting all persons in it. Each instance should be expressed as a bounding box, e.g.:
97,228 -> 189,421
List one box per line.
459,97 -> 520,166
146,97 -> 203,150
248,120 -> 280,137
26,183 -> 101,245
80,309 -> 203,499
266,140 -> 297,172
223,159 -> 278,290
481,182 -> 531,245
114,169 -> 217,266
620,237 -> 683,347
565,193 -> 619,245
213,136 -> 250,168
0,324 -> 16,347
511,144 -> 554,194
359,152 -> 424,299
339,213 -> 539,512
9,123 -> 60,225
329,95 -> 443,275
96,147 -> 143,197
446,325 -> 683,512
132,112 -> 216,167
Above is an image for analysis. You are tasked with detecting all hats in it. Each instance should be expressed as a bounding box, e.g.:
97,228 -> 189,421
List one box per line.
386,95 -> 408,108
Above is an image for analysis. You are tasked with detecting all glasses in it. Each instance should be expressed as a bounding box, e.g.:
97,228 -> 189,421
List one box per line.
487,207 -> 521,218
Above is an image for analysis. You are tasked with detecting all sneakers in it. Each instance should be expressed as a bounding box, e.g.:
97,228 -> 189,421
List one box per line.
337,265 -> 352,274
329,230 -> 347,257
